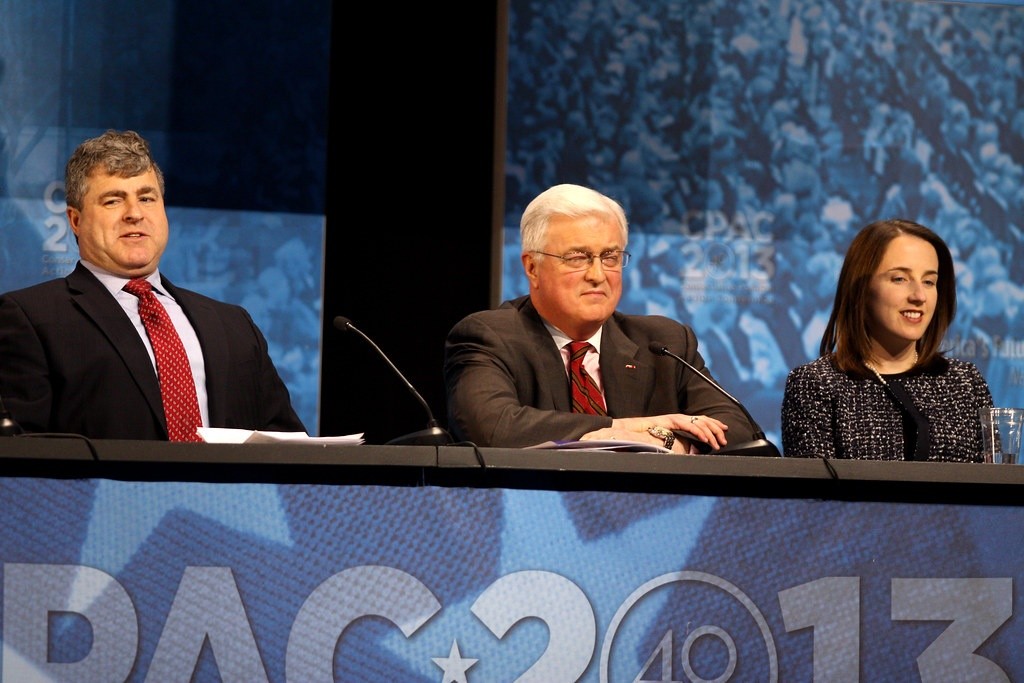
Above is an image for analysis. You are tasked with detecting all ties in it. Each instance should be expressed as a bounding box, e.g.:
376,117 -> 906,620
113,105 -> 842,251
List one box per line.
565,342 -> 607,416
122,278 -> 203,444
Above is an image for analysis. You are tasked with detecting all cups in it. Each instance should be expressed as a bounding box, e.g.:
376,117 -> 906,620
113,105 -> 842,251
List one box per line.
976,408 -> 1024,464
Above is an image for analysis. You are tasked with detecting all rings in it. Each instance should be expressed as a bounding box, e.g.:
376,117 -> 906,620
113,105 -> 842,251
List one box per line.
610,436 -> 615,439
691,416 -> 698,423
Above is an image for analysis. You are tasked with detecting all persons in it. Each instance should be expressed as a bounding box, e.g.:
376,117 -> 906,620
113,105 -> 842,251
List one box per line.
444,184 -> 753,454
780,221 -> 1002,464
0,129 -> 309,441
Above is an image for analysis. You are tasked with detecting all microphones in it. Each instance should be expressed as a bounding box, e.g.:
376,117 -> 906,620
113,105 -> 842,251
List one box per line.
647,340 -> 782,459
334,316 -> 454,447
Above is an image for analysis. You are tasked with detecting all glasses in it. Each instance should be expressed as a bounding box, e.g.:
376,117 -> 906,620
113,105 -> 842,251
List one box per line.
529,250 -> 632,271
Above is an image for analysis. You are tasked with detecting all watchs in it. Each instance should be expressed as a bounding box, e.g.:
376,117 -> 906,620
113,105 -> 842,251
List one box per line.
648,425 -> 675,451
